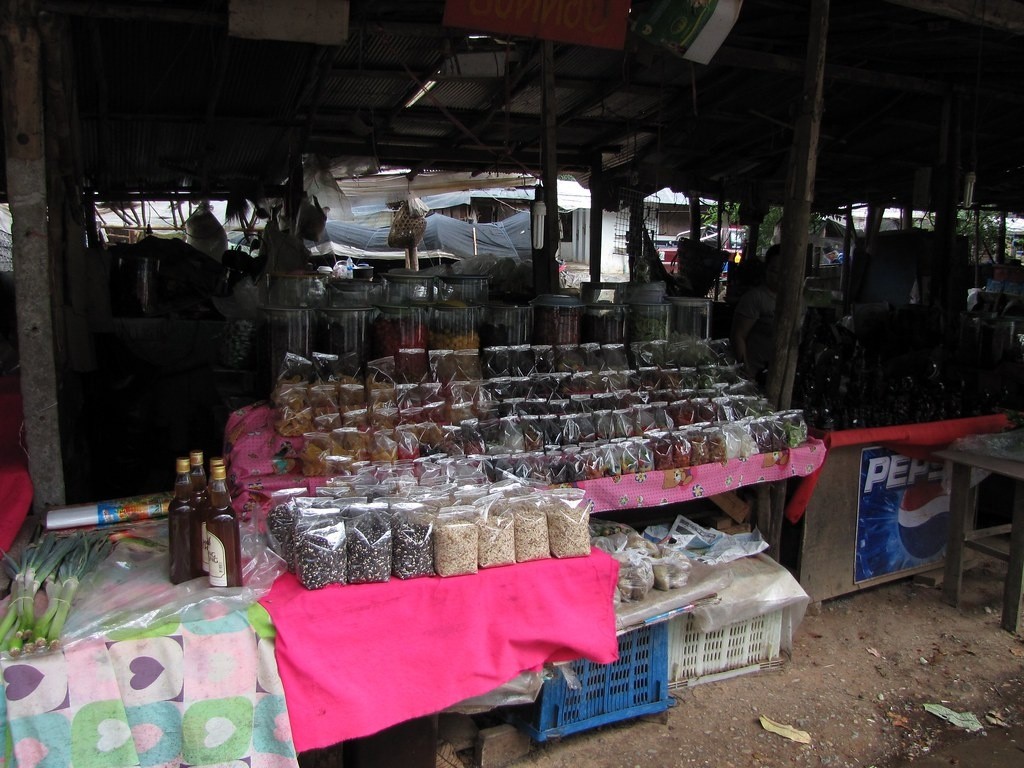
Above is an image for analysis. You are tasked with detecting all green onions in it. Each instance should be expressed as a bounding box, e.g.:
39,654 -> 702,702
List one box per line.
0,526 -> 168,657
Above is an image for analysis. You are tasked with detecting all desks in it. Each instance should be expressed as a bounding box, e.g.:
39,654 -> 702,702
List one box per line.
932,447 -> 1024,634
0,519 -> 619,768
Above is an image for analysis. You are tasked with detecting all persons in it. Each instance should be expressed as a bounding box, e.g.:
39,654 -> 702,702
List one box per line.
731,244 -> 809,378
0,277 -> 19,371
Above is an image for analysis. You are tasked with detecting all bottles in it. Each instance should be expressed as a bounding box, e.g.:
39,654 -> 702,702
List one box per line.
189,452 -> 207,511
206,465 -> 243,589
199,458 -> 232,577
168,456 -> 198,586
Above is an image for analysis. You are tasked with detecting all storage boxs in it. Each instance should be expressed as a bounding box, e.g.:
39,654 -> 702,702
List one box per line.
500,551 -> 676,740
667,555 -> 799,688
